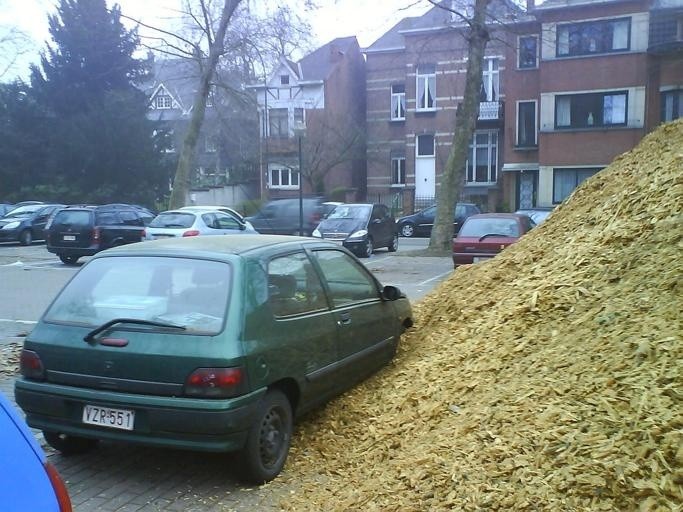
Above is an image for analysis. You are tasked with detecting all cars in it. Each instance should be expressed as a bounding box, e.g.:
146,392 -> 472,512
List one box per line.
13,233 -> 415,490
0,388 -> 72,511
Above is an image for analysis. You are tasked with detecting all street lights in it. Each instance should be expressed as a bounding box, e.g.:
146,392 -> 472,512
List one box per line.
292,119 -> 309,237
